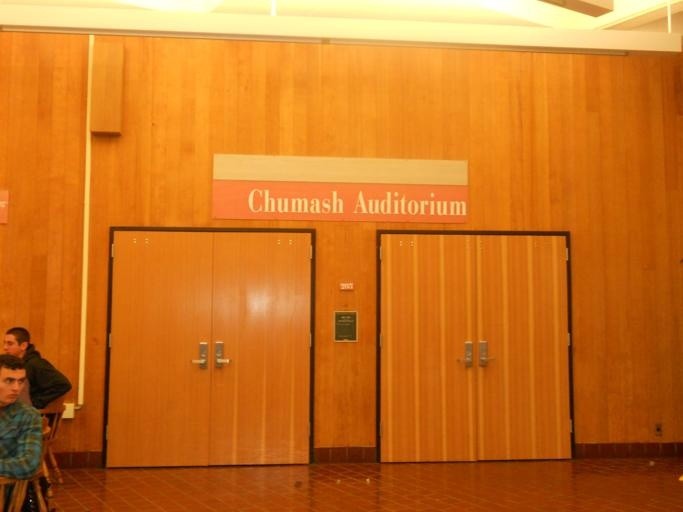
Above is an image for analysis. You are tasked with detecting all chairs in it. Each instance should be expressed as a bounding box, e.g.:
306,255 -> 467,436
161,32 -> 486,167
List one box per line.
0,417 -> 52,512
39,395 -> 66,485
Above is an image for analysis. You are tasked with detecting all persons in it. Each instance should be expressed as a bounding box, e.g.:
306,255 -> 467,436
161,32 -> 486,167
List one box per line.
2,326 -> 72,410
0,354 -> 43,512
